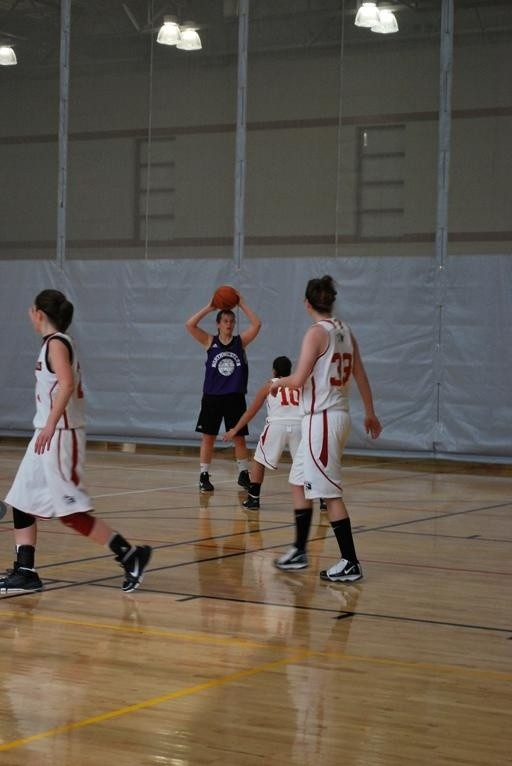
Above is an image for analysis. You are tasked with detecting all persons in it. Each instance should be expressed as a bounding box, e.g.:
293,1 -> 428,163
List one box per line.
1,289 -> 151,606
263,273 -> 383,587
184,291 -> 263,493
221,356 -> 329,514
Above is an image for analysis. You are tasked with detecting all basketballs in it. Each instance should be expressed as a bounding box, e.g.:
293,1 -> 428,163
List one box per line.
214,287 -> 237,311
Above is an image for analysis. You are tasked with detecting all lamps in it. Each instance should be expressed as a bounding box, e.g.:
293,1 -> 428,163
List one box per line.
156,11 -> 205,52
1,41 -> 19,67
351,0 -> 402,36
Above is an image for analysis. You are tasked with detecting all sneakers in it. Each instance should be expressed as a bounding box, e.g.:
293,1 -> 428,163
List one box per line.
198,471 -> 214,491
271,547 -> 308,569
237,469 -> 251,490
320,498 -> 328,511
114,544 -> 153,593
0,560 -> 44,594
242,488 -> 260,510
319,559 -> 363,583
